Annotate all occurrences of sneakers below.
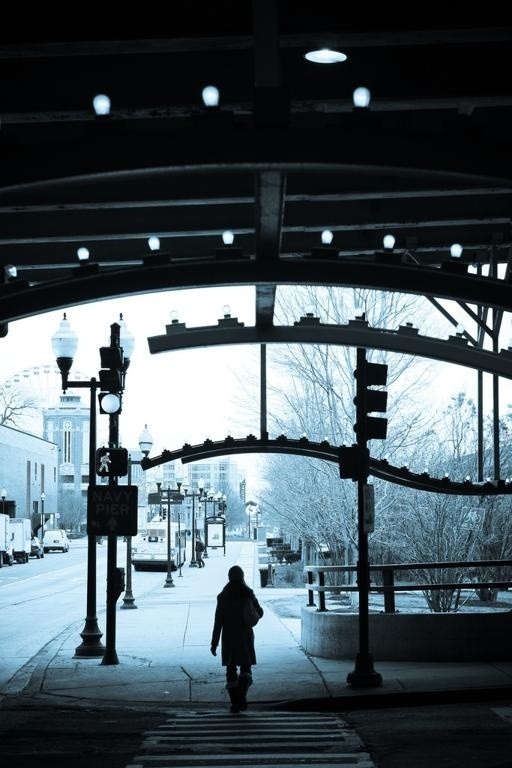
[197,563,206,569]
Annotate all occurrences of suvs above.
[42,529,70,553]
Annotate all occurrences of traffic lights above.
[95,448,128,476]
[99,345,123,415]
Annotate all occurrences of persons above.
[211,564,264,713]
[195,536,205,568]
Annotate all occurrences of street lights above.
[1,487,7,513]
[40,491,47,538]
[120,423,228,609]
[52,310,130,660]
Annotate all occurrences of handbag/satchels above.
[244,586,259,628]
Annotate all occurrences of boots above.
[238,673,254,712]
[226,679,242,713]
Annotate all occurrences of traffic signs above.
[363,484,374,533]
[459,507,485,531]
[87,484,138,537]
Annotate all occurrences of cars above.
[30,536,44,559]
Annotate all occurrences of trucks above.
[0,513,31,569]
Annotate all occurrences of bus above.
[130,521,192,572]
